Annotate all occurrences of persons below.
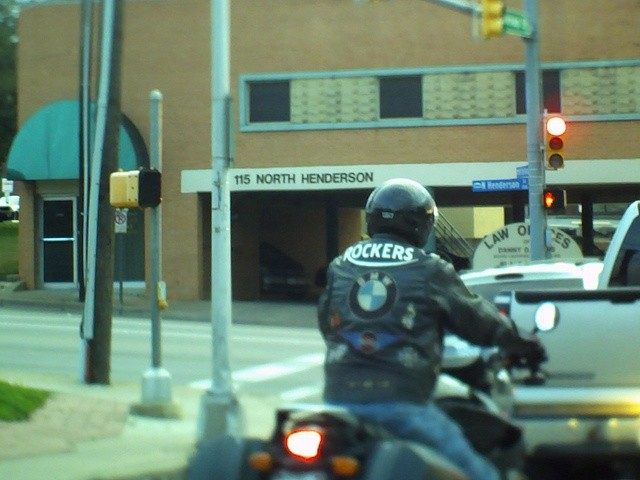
[316,179,547,480]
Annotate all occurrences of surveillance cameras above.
[1,177,14,205]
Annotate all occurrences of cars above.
[441,255,604,373]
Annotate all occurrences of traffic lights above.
[481,0,504,38]
[543,187,558,210]
[543,112,566,170]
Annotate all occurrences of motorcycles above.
[244,301,562,480]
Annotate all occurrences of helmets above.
[366,177,437,247]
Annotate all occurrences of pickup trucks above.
[489,199,640,458]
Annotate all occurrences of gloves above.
[506,326,548,370]
